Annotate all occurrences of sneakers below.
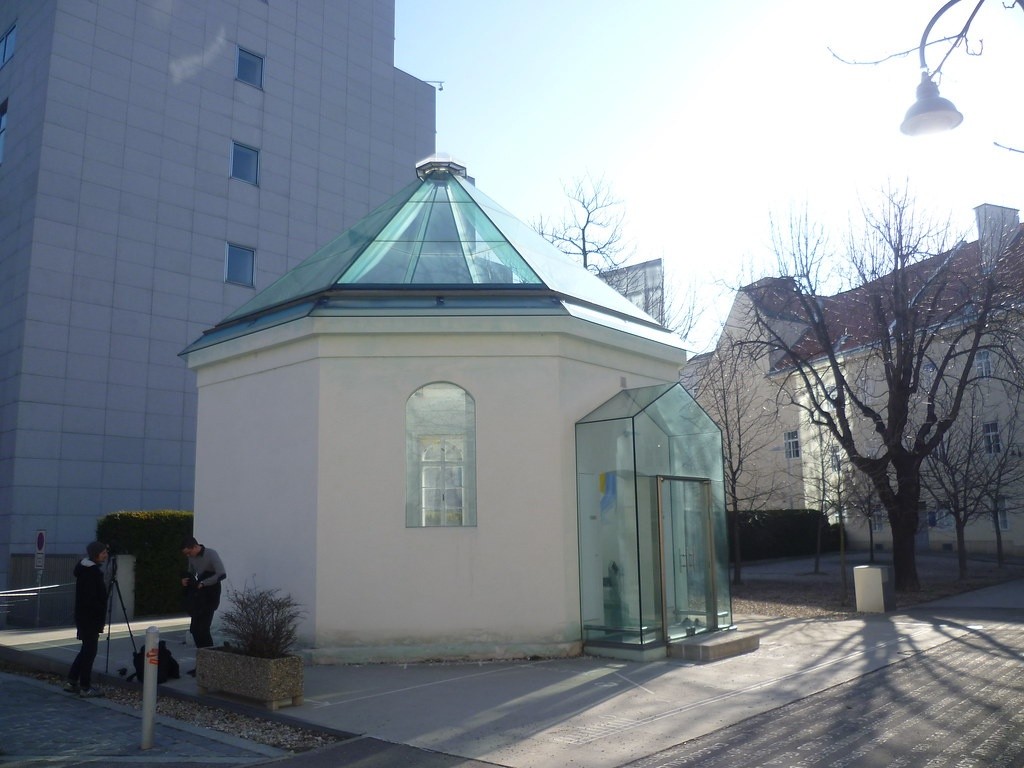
[63,683,81,692]
[80,688,104,698]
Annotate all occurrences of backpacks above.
[125,640,180,685]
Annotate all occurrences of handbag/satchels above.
[180,577,206,616]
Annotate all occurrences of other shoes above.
[187,669,196,677]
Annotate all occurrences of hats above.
[86,541,106,559]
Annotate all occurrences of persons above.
[180,536,226,678]
[63,541,105,697]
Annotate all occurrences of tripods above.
[107,577,137,674]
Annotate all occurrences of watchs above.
[201,581,204,586]
